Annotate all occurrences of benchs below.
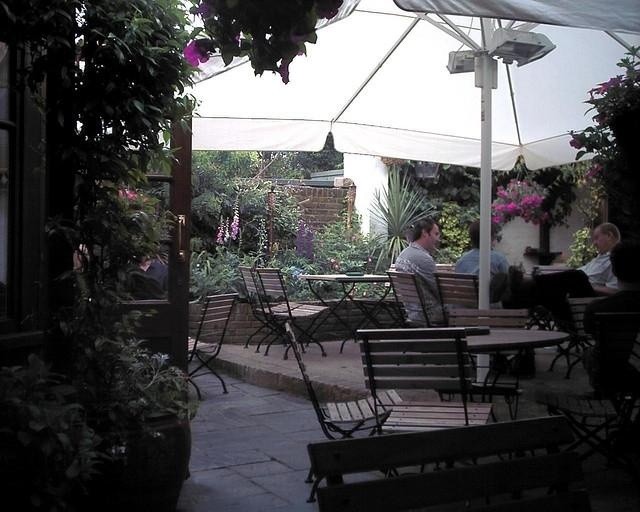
[306,416,591,512]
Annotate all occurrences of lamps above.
[488,28,556,67]
[446,50,474,74]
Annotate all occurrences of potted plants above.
[0,183,198,512]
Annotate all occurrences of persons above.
[453,216,514,310]
[582,238,640,400]
[395,218,450,328]
[484,221,623,303]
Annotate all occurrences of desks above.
[304,273,391,355]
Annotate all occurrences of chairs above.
[188,293,239,399]
[356,268,640,491]
[239,265,330,361]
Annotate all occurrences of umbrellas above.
[75,0,640,390]
